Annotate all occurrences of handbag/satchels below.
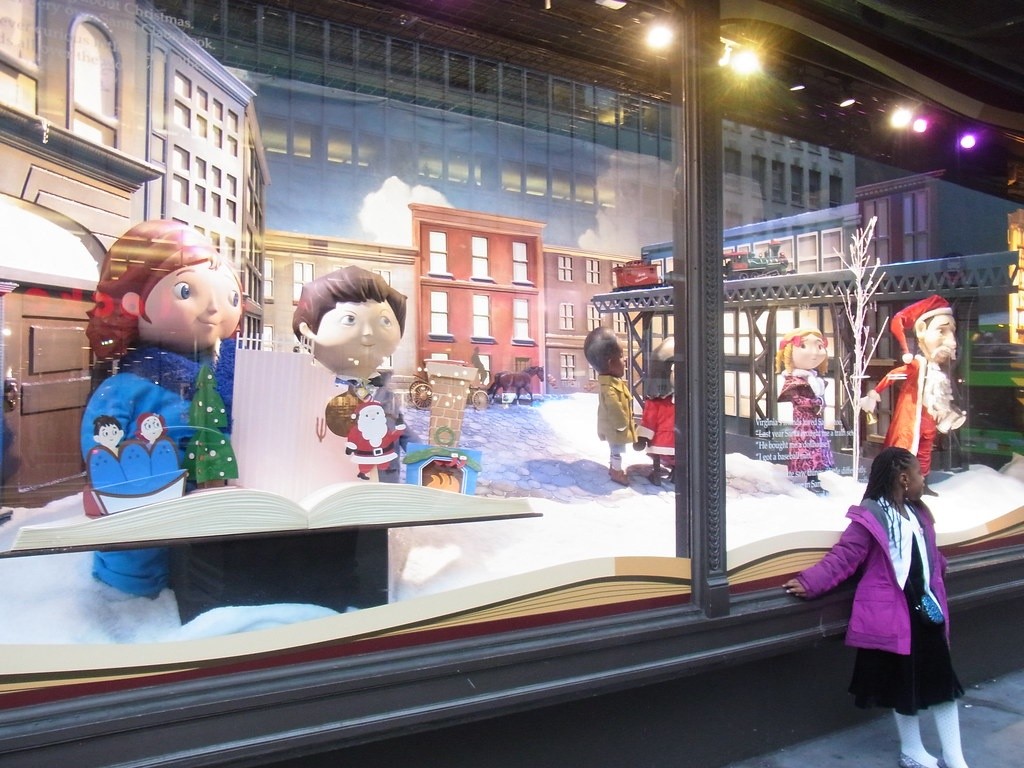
[922,594,943,624]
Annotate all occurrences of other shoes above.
[900,752,947,768]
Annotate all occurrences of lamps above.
[786,70,806,91]
[836,81,856,107]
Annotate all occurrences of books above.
[1,479,546,561]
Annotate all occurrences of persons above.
[584,325,639,486]
[632,335,676,487]
[292,263,425,482]
[78,218,244,603]
[774,325,833,497]
[858,294,968,497]
[783,445,970,768]
